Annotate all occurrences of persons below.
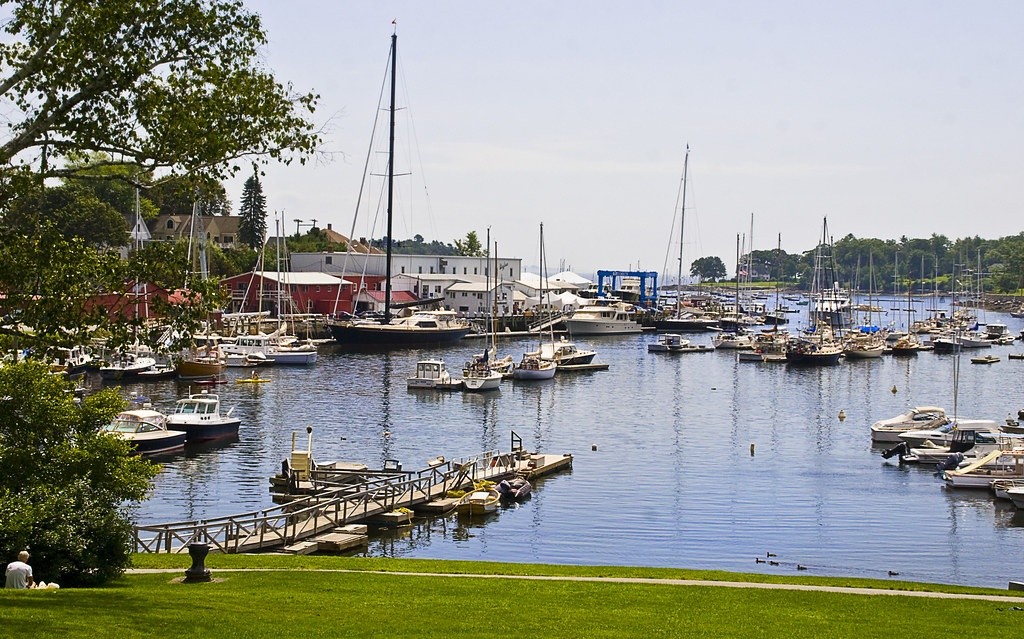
[5,551,36,588]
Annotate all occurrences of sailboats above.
[643,144,1024,365]
[0,170,317,404]
[465,221,610,391]
[325,19,471,349]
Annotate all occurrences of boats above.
[872,405,1024,513]
[456,476,535,516]
[406,358,463,391]
[564,295,649,337]
[93,386,241,464]
[268,427,371,503]
